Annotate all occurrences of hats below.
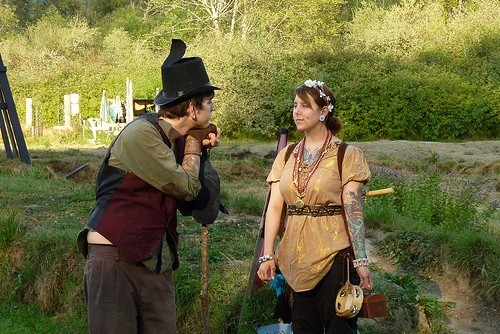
[155,39,222,105]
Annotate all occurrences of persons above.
[257,80,373,334]
[75,38,229,334]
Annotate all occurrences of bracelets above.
[258,255,274,262]
[352,258,369,268]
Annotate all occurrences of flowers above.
[304,78,334,113]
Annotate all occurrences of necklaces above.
[292,129,332,209]
